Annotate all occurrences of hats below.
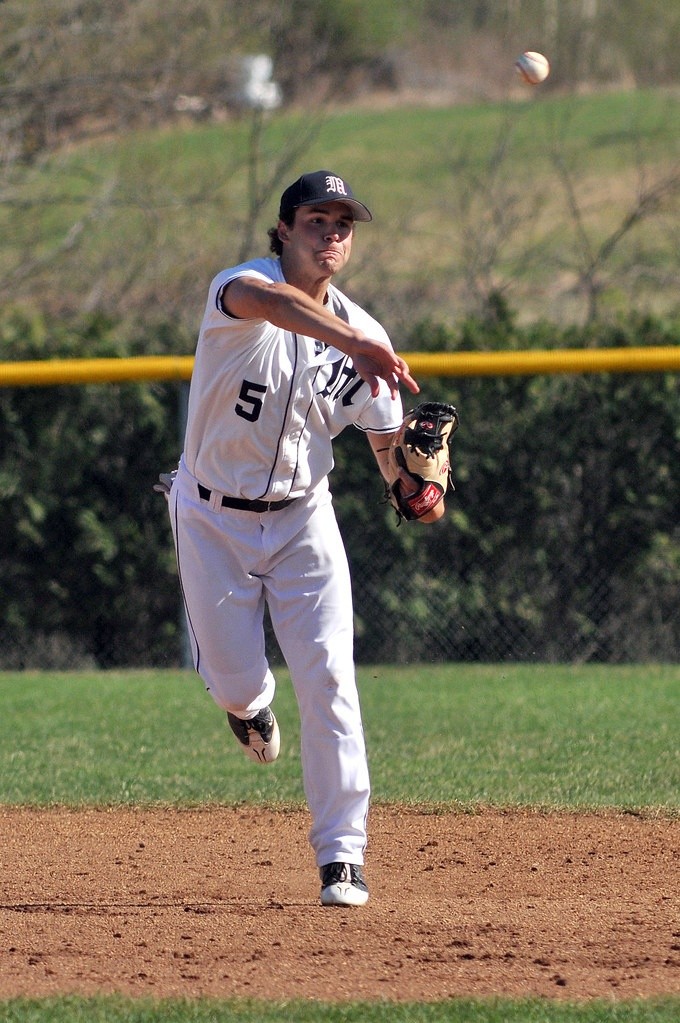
[280,171,374,222]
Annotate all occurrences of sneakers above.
[226,706,281,763]
[320,861,371,906]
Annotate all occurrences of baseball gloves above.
[374,400,460,529]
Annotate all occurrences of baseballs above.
[515,51,551,85]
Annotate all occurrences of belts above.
[198,482,296,514]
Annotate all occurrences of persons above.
[153,170,455,906]
